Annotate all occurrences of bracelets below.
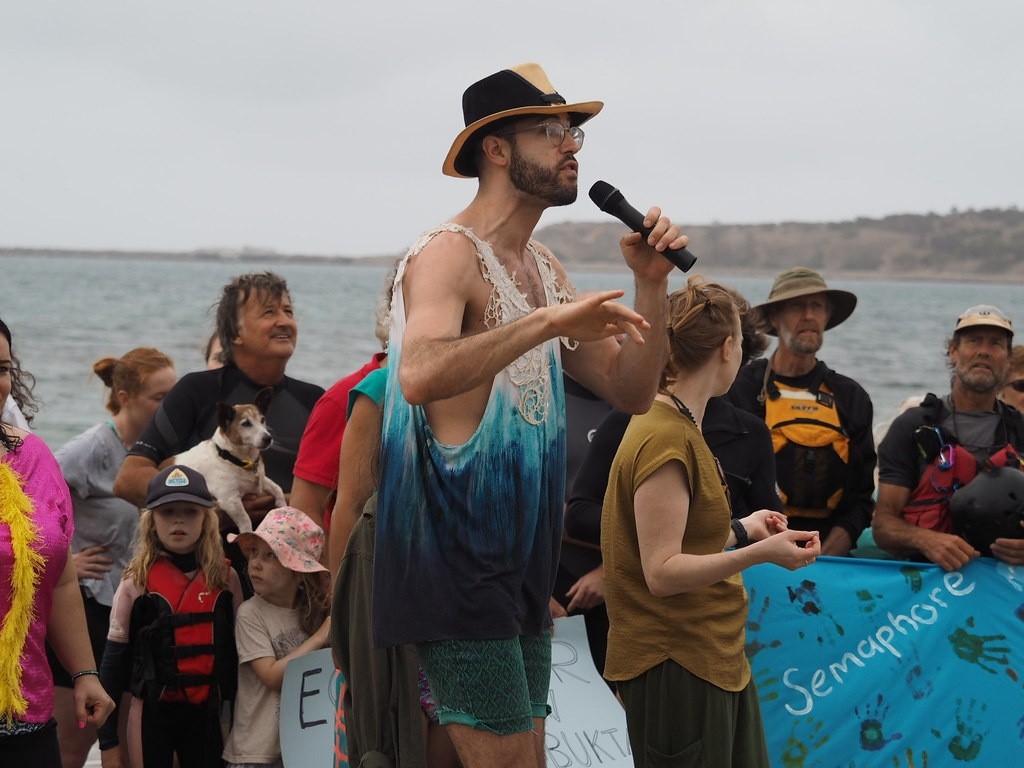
[71,670,100,682]
[731,517,749,549]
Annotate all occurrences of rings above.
[805,560,808,567]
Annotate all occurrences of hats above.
[953,304,1014,334]
[228,506,331,572]
[442,62,604,179]
[750,267,857,337]
[146,465,212,511]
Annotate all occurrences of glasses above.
[498,122,585,151]
[1004,379,1024,392]
[952,396,1008,455]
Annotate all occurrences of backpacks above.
[754,380,850,532]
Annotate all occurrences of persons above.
[0,64,1024,768]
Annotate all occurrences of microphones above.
[590,180,697,272]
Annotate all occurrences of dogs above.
[158,386,287,535]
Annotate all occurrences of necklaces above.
[666,389,733,517]
